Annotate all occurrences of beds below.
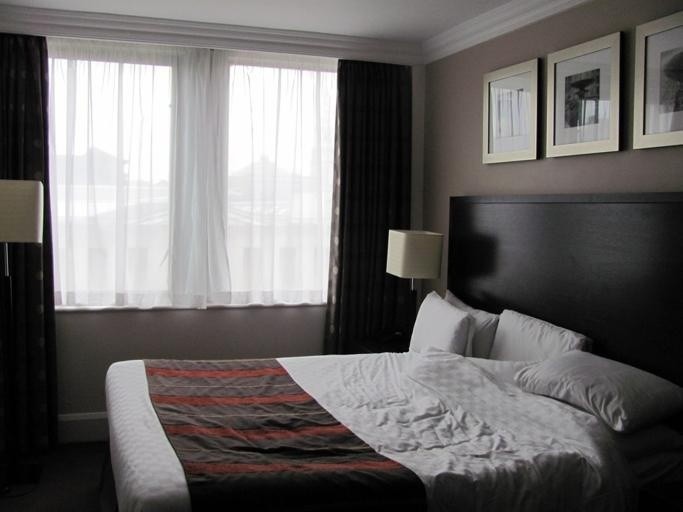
[104,193,683,510]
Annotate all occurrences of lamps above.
[387,228,443,315]
[0,178,44,309]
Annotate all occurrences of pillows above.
[408,290,473,356]
[516,350,682,431]
[443,291,499,359]
[487,309,588,368]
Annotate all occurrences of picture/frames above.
[545,32,621,158]
[631,8,683,150]
[482,58,539,164]
